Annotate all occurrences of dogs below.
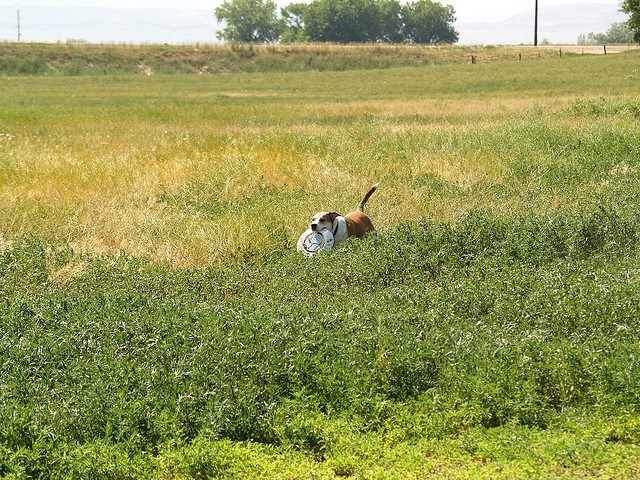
[311,183,381,248]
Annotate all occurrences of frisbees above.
[297,229,334,256]
